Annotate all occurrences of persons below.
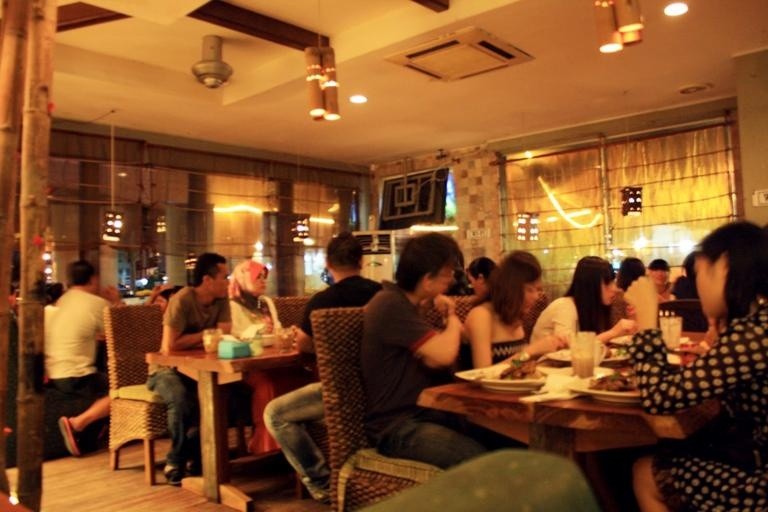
[531,257,636,361]
[40,284,67,386]
[647,258,675,302]
[618,255,662,332]
[468,255,497,294]
[46,259,111,456]
[621,220,768,512]
[358,232,490,472]
[226,259,311,453]
[459,250,569,388]
[670,252,708,333]
[261,233,384,507]
[146,252,233,487]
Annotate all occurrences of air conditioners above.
[351,229,394,283]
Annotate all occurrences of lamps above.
[46,280,52,284]
[44,267,52,274]
[289,149,311,238]
[102,110,126,242]
[307,81,325,121]
[156,216,167,234]
[324,86,340,123]
[46,276,53,279]
[118,172,128,178]
[664,2,689,17]
[621,91,643,217]
[322,1,338,84]
[213,151,603,234]
[41,252,52,261]
[593,0,624,54]
[623,33,643,44]
[304,0,322,88]
[612,0,644,33]
[184,252,199,270]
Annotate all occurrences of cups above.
[203,329,223,352]
[276,329,293,352]
[572,332,607,378]
[660,317,682,350]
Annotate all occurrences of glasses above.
[440,270,455,278]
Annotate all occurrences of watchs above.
[518,350,531,360]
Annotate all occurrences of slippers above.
[60,416,82,455]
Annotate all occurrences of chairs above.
[310,306,446,512]
[521,292,548,344]
[104,304,179,487]
[260,296,309,327]
[353,450,601,512]
[657,298,708,332]
[424,295,477,333]
[296,419,325,499]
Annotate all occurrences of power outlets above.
[752,189,768,207]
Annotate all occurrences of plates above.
[570,386,641,402]
[241,335,276,346]
[544,349,631,362]
[609,335,633,345]
[454,367,547,392]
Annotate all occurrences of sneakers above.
[186,462,200,475]
[165,467,182,484]
